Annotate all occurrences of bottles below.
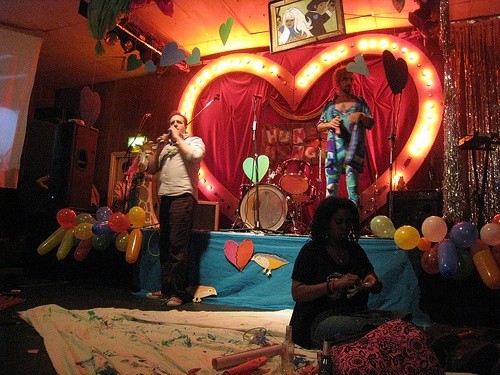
[319,339,333,375]
[280,324,297,375]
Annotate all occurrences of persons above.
[316,66,374,239]
[290,195,463,366]
[144,114,206,307]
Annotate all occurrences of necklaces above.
[329,243,349,263]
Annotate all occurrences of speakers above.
[192,200,220,232]
[49,122,99,209]
[387,189,443,237]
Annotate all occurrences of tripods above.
[220,98,284,234]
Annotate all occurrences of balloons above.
[369,214,500,290]
[38,206,147,263]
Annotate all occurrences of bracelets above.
[326,279,336,295]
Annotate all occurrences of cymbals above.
[303,133,328,148]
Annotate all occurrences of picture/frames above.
[107,151,150,207]
[268,0,346,53]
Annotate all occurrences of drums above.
[288,185,317,202]
[279,159,312,194]
[239,183,296,234]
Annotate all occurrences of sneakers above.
[146,291,164,299]
[161,297,183,306]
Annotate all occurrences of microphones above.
[253,94,262,99]
[144,113,151,117]
